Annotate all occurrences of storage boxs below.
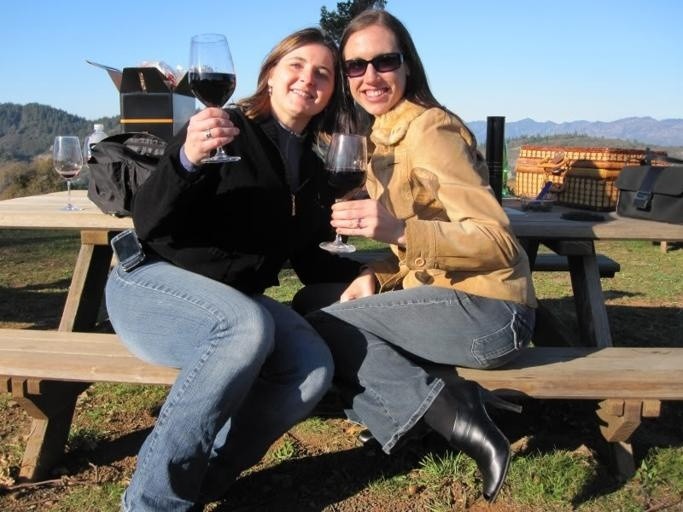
[84,59,201,153]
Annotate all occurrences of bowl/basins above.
[519,192,558,211]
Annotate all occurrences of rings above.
[355,218,362,228]
[205,130,212,140]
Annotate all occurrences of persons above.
[102,29,362,512]
[293,10,541,502]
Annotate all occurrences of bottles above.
[84,123,109,164]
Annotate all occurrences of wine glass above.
[52,134,86,213]
[189,33,242,165]
[319,132,368,253]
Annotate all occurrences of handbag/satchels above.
[87,131,169,217]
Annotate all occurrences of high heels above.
[448,380,523,505]
[359,422,430,449]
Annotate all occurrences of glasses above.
[342,52,404,78]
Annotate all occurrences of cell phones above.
[110,229,147,273]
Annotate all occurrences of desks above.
[0,190,683,461]
